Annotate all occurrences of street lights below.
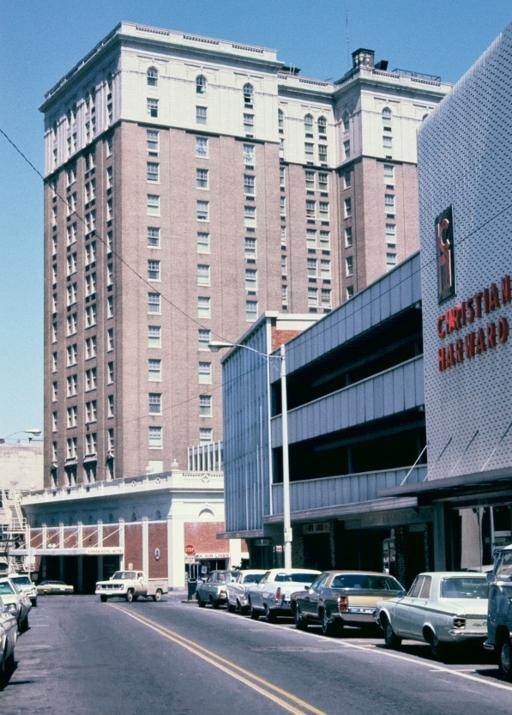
[208,340,293,568]
[0,428,42,443]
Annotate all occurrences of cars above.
[0,574,38,675]
[37,580,75,595]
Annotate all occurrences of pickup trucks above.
[95,570,168,603]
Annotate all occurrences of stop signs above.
[184,544,196,557]
